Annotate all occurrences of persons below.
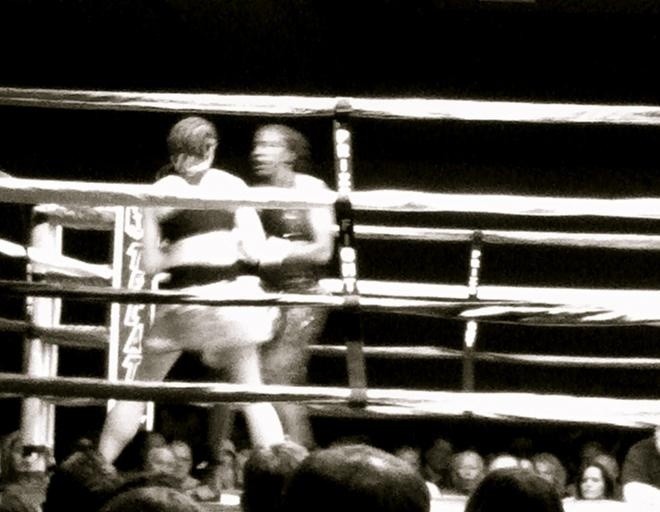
[209,122,337,454]
[1,428,659,512]
[96,113,311,468]
